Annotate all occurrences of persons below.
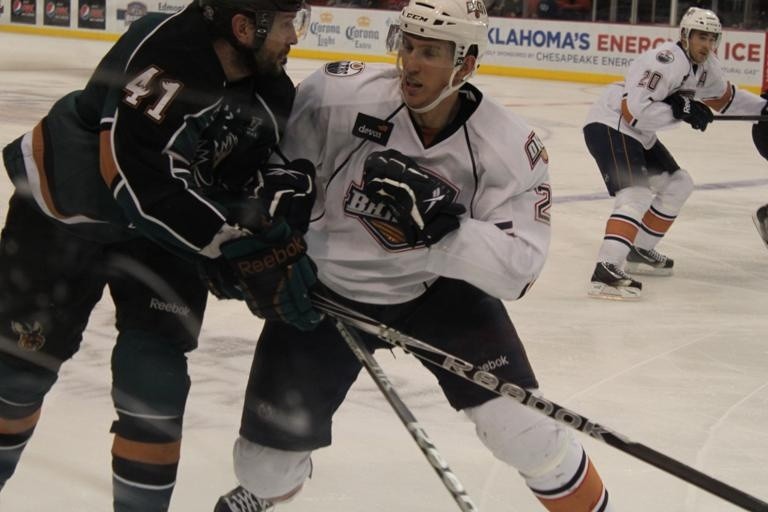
[0,0,322,512]
[213,0,616,512]
[583,8,768,291]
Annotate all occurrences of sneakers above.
[214,482,302,512]
[592,262,641,290]
[757,203,767,244]
[627,245,673,268]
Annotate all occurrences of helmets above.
[679,7,722,51]
[198,0,301,52]
[395,0,490,91]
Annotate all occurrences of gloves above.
[664,96,713,131]
[362,149,466,247]
[198,158,324,332]
